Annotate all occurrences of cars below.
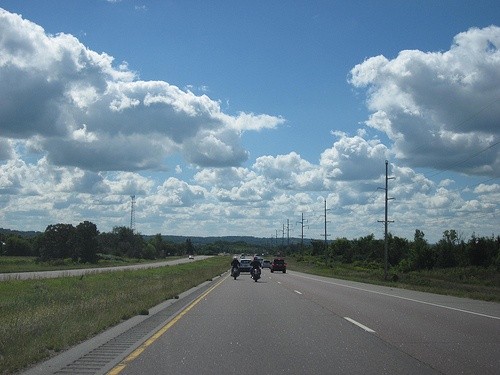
[239,255,252,271]
[258,259,271,269]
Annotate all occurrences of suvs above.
[271,259,287,273]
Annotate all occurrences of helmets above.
[254,257,258,261]
[234,257,237,260]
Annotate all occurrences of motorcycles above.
[231,263,240,280]
[250,264,261,282]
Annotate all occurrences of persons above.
[231,256,240,274]
[250,257,261,279]
